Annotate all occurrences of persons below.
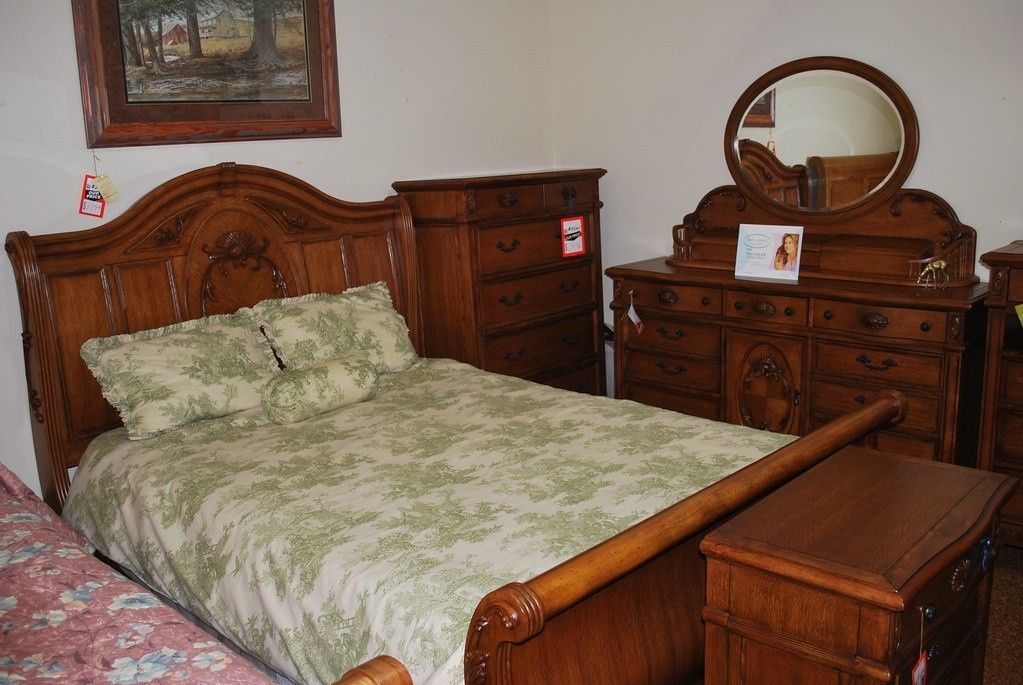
[769,233,799,272]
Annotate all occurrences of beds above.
[5,161,910,684]
[1,465,412,684]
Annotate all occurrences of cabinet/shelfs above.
[391,168,608,396]
[700,444,1020,684]
[604,184,989,467]
[975,241,1023,551]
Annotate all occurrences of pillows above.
[254,280,427,374]
[261,357,380,423]
[80,307,283,442]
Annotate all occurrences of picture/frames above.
[71,0,343,150]
[741,88,776,128]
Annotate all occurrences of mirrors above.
[723,56,919,224]
